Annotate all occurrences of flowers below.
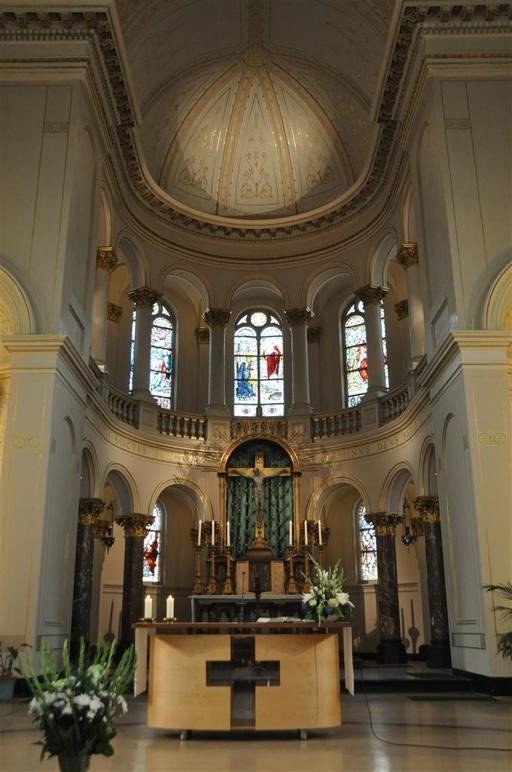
[300,553,356,628]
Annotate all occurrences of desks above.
[137,621,354,740]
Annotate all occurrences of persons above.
[230,467,289,528]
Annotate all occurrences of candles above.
[197,520,201,545]
[304,520,308,546]
[166,595,175,618]
[288,520,293,545]
[318,520,322,545]
[144,594,152,618]
[211,520,215,545]
[227,522,231,546]
